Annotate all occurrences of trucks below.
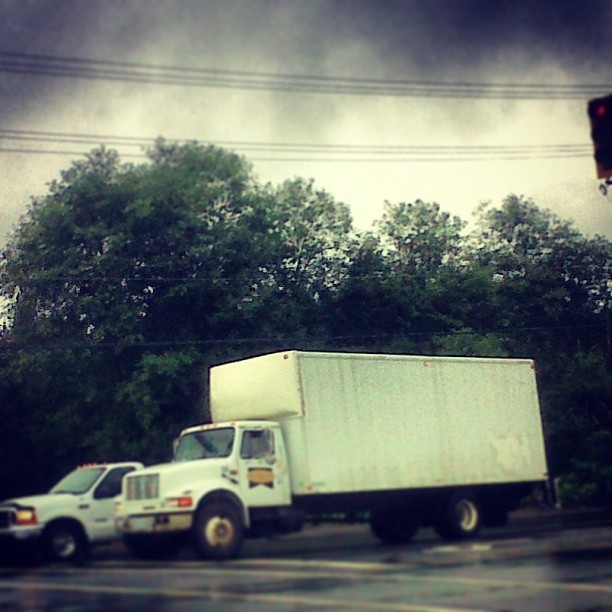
[119,350,548,562]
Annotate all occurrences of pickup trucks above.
[0,462,196,562]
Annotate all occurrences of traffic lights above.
[589,93,612,178]
